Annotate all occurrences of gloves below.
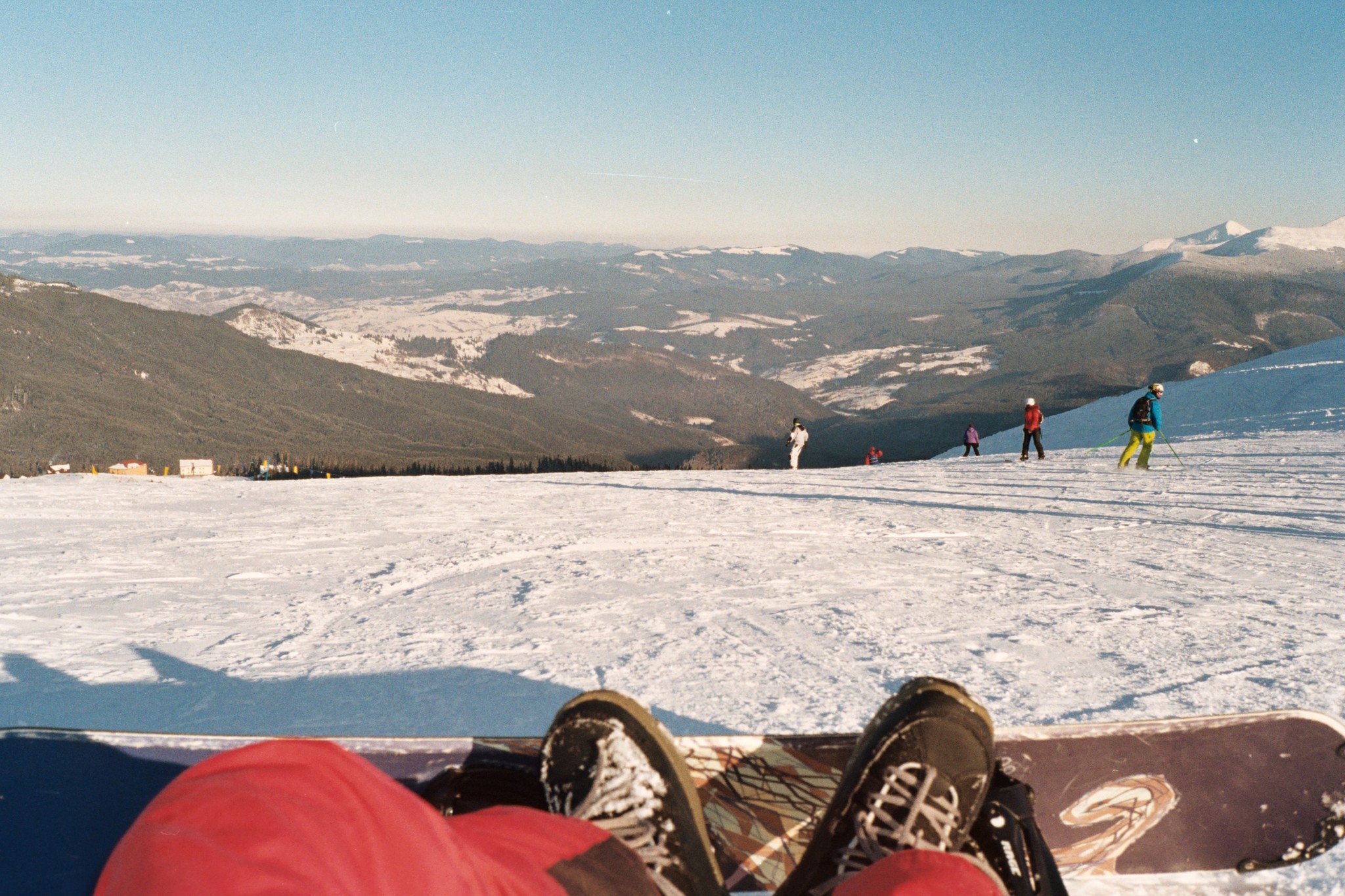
[785,443,788,447]
[964,443,966,446]
[804,445,807,448]
[976,443,979,448]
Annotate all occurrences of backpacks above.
[1129,395,1157,425]
[1036,409,1044,424]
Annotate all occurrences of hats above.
[1148,383,1164,395]
[969,424,974,428]
[793,418,799,424]
[1026,398,1035,405]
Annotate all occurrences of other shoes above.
[1020,454,1029,460]
[1039,455,1044,460]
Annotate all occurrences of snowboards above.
[0,698,1345,896]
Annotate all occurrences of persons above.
[866,447,883,465]
[963,424,980,457]
[1020,398,1046,461]
[98,678,1010,896]
[785,418,808,469]
[1117,382,1164,471]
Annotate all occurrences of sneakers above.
[808,677,995,895]
[538,691,728,896]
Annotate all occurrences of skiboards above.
[1003,458,1052,465]
[1110,463,1192,474]
